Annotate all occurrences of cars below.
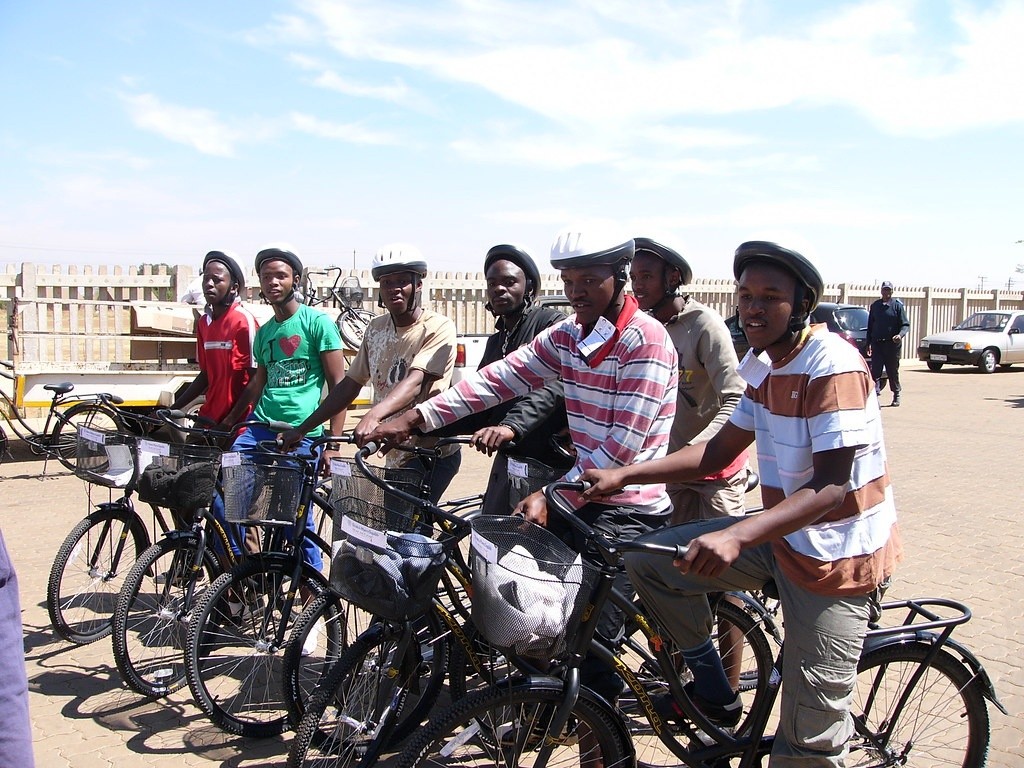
[915,309,1024,374]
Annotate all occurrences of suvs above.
[721,301,890,391]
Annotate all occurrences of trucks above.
[8,273,375,445]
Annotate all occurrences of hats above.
[881,281,894,291]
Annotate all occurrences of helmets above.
[733,241,824,313]
[204,251,245,294]
[484,244,542,301]
[550,228,636,270]
[254,248,302,279]
[632,236,693,285]
[371,251,428,282]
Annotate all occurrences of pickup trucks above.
[448,293,578,389]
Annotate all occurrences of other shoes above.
[636,679,742,728]
[153,562,204,583]
[493,713,578,746]
[222,596,266,624]
[892,392,901,406]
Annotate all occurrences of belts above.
[874,338,891,342]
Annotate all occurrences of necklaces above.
[501,312,522,358]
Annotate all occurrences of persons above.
[212,249,348,621]
[1004,316,1024,333]
[145,250,262,582]
[272,240,461,630]
[574,241,903,768]
[392,242,577,670]
[865,281,911,407]
[357,230,676,768]
[630,233,749,744]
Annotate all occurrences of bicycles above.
[0,356,126,476]
[45,391,546,761]
[297,266,379,354]
[393,479,1013,768]
[285,439,785,768]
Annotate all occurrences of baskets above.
[329,496,457,622]
[76,421,143,488]
[135,436,224,509]
[339,276,364,304]
[470,514,599,663]
[220,451,307,526]
[330,457,422,518]
